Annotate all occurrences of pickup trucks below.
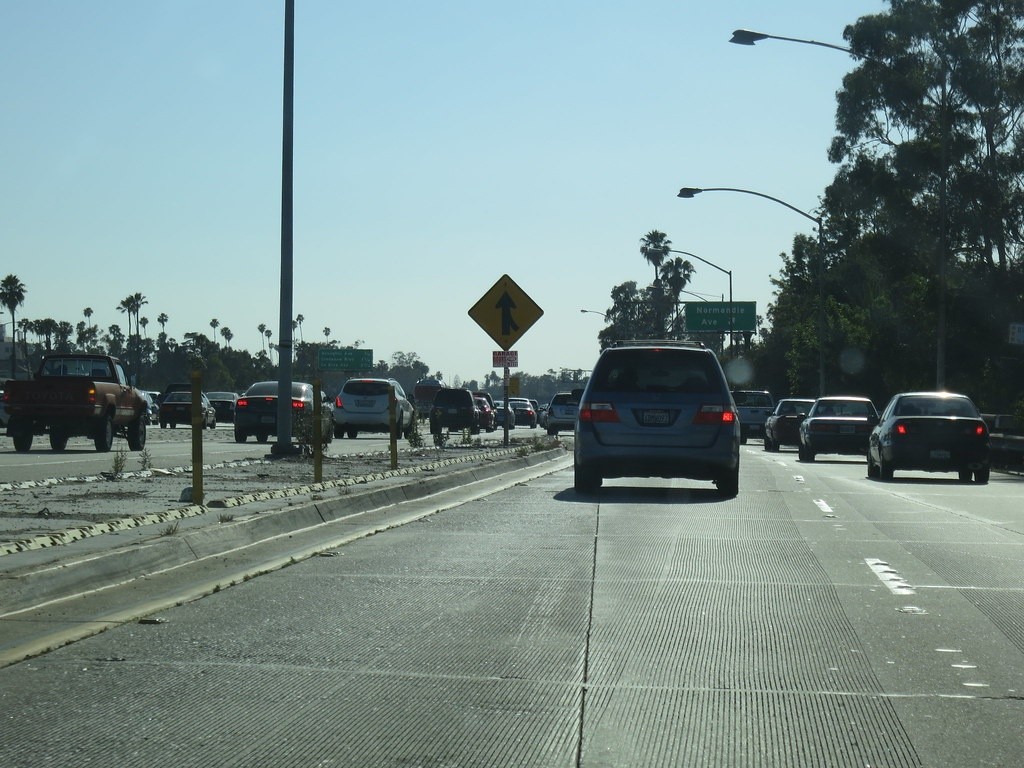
[730,390,777,444]
[3,351,149,454]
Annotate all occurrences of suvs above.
[572,340,748,499]
[429,387,481,435]
[331,376,415,440]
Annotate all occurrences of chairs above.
[754,397,766,407]
[821,407,836,416]
[607,369,634,392]
[900,403,922,415]
[781,406,796,416]
[734,394,748,406]
[92,368,106,377]
[851,406,867,416]
[50,364,67,376]
[676,376,711,393]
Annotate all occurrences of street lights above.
[649,246,732,394]
[677,186,827,398]
[729,29,947,392]
[646,285,725,367]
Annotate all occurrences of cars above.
[205,391,240,423]
[0,377,161,428]
[763,398,817,453]
[159,389,216,430]
[234,381,331,444]
[866,391,994,484]
[796,396,880,463]
[470,390,579,436]
